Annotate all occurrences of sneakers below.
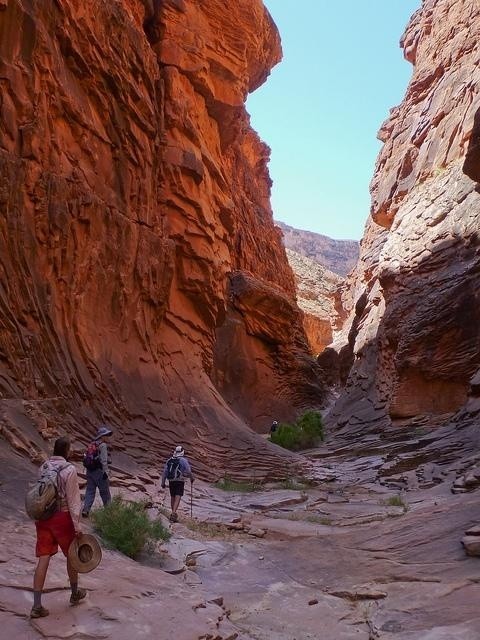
[170,512,178,521]
[30,605,49,617]
[70,589,86,604]
[82,509,90,518]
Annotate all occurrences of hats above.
[273,421,277,425]
[69,534,102,573]
[173,446,184,457]
[95,428,111,440]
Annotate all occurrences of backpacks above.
[25,461,72,520]
[83,441,103,468]
[167,457,182,479]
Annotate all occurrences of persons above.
[268,418,279,435]
[160,444,196,525]
[29,435,88,620]
[80,427,114,519]
